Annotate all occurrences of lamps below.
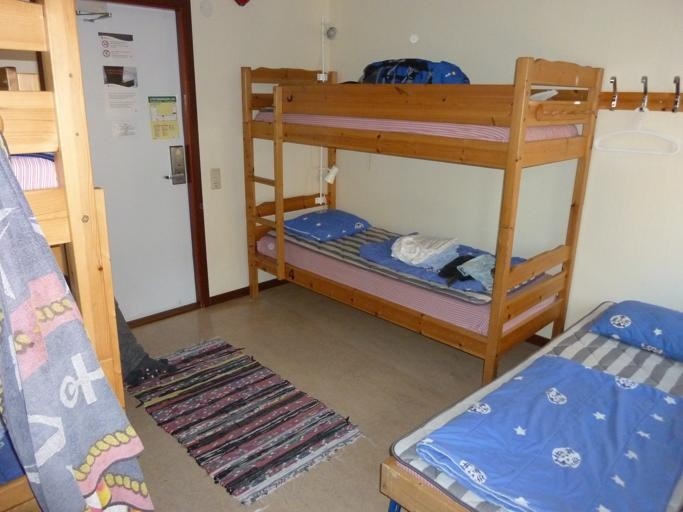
[318,165,339,204]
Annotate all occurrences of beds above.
[1,0,123,512]
[239,45,603,388]
[378,279,683,512]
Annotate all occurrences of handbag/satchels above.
[359,58,470,84]
[391,233,461,271]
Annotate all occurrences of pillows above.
[283,209,372,242]
[589,299,683,364]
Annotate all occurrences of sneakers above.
[127,358,176,384]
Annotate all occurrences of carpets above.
[124,336,365,508]
